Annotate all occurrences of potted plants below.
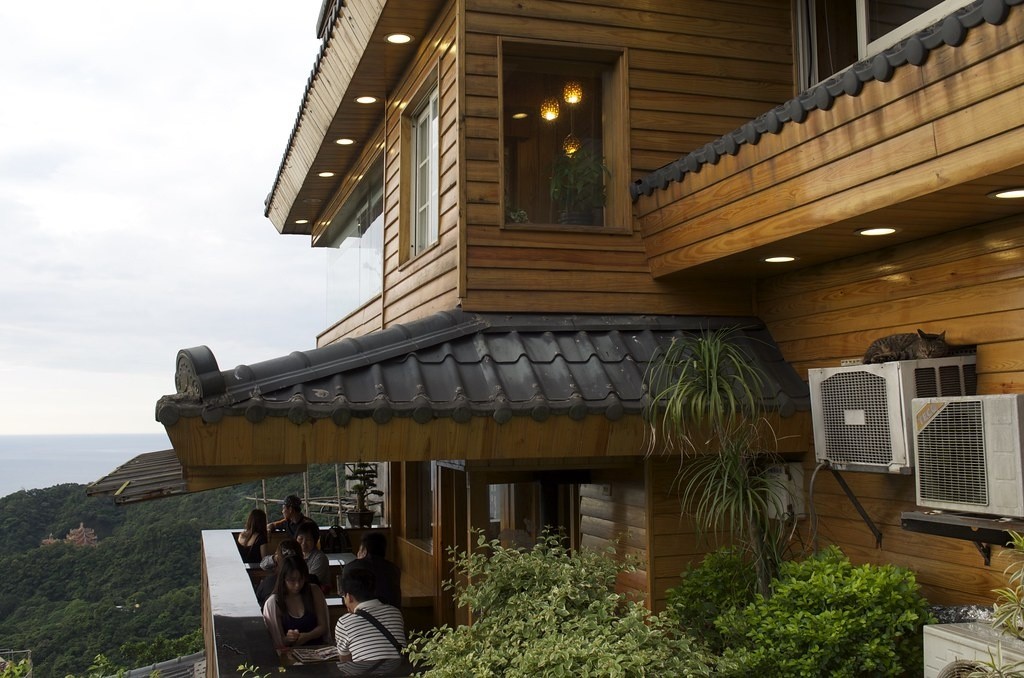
[338,461,384,529]
[544,148,612,226]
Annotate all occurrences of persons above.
[256,539,320,609]
[264,554,331,647]
[335,568,407,662]
[338,532,400,609]
[260,522,330,584]
[336,660,402,678]
[266,496,321,551]
[234,509,267,563]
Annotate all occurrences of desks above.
[244,551,358,584]
[281,644,347,678]
[324,597,348,621]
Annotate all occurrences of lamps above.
[541,78,584,158]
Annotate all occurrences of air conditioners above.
[908,393,1024,521]
[922,622,1024,678]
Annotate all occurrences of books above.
[292,646,338,663]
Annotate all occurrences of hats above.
[276,494,302,508]
